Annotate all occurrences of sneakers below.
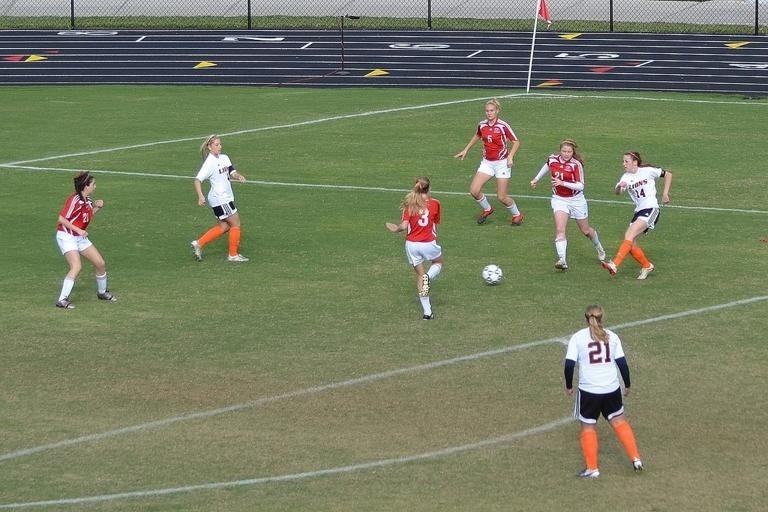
[633,458,644,472]
[227,254,249,262]
[96,289,116,301]
[190,240,202,261]
[422,312,435,321]
[56,298,76,309]
[420,273,431,297]
[575,468,599,480]
[477,208,653,278]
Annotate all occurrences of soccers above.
[482,264,502,285]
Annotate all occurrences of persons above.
[54,169,118,309]
[187,133,252,264]
[599,149,675,281]
[529,138,606,270]
[563,302,646,478]
[453,98,524,228]
[385,176,443,322]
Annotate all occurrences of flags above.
[537,0,552,29]
